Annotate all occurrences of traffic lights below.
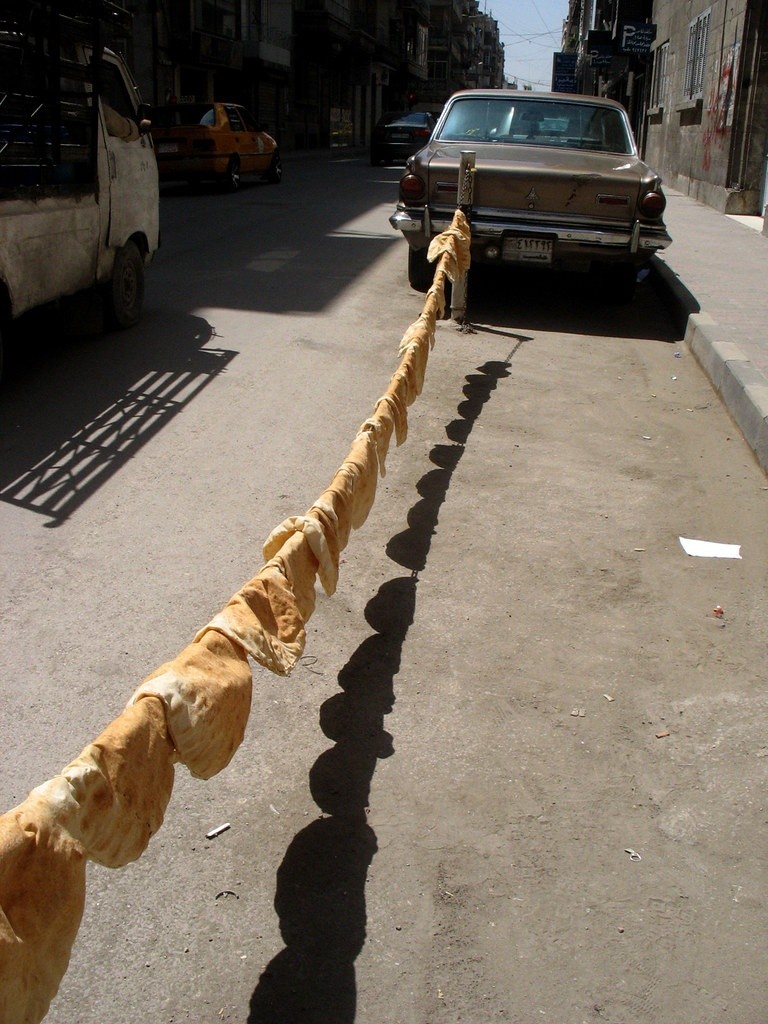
[409,94,417,107]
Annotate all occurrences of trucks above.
[1,0,177,333]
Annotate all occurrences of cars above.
[388,87,673,306]
[152,102,283,194]
[369,110,437,166]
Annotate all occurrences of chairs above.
[567,118,591,136]
